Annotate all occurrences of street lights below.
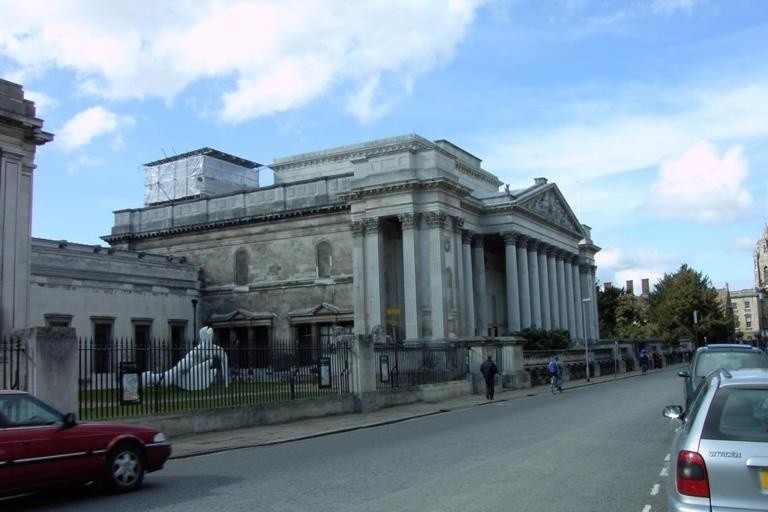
[190,298,198,350]
[580,297,592,383]
[727,330,733,343]
[703,336,707,345]
[758,286,767,344]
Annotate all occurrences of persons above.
[548,356,564,392]
[480,355,499,400]
[638,350,648,372]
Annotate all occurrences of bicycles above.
[639,364,647,375]
[549,367,565,396]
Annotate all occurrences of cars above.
[661,365,767,512]
[677,342,767,408]
[0,386,175,504]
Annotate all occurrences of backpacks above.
[550,363,556,374]
